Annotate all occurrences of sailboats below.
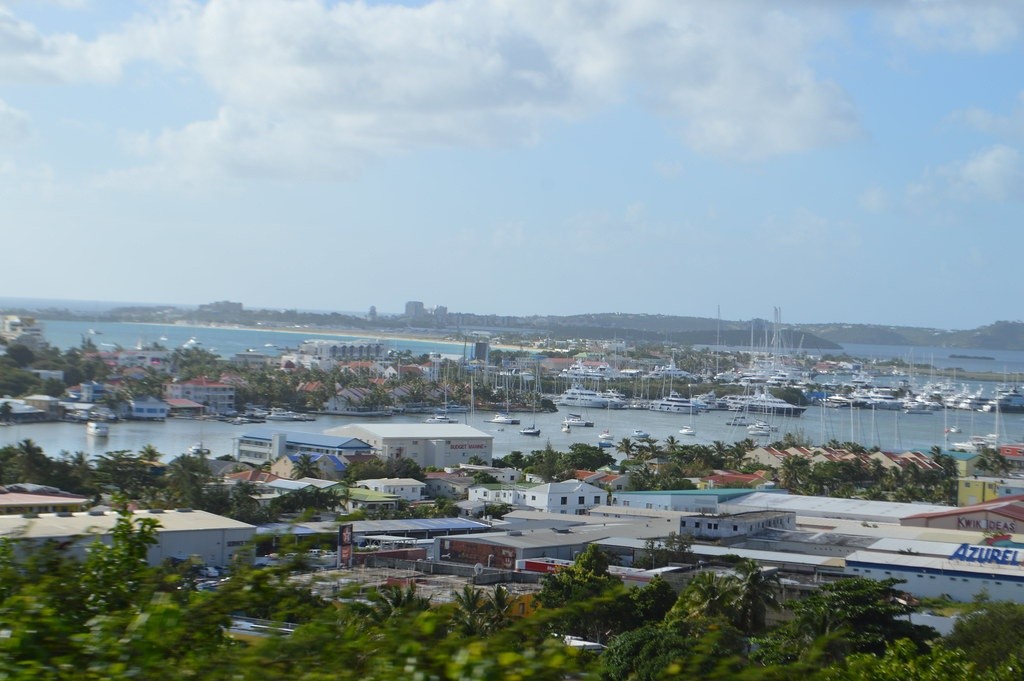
[726,375,780,437]
[562,376,594,427]
[519,384,540,435]
[679,394,697,436]
[599,399,615,440]
[483,377,520,425]
[423,377,459,424]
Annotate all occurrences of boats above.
[221,408,316,421]
[818,350,1024,415]
[550,307,807,416]
[631,428,649,438]
[562,423,571,432]
[591,441,614,449]
[87,422,109,437]
